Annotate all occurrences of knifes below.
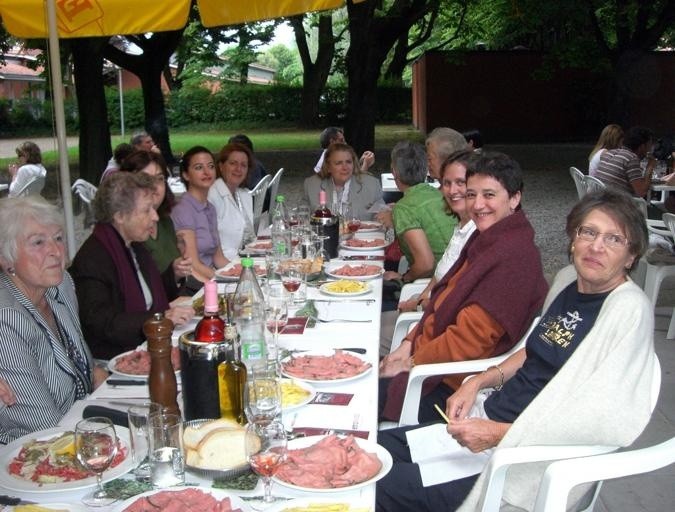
[106,379,184,386]
[1,495,37,506]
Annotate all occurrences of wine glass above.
[72,414,121,507]
[244,420,289,510]
[242,377,282,451]
[265,300,289,352]
[253,200,354,309]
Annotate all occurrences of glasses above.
[576,223,631,249]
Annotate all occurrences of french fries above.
[247,380,312,407]
[279,503,371,512]
[9,504,71,511]
[324,278,366,294]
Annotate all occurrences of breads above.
[181,418,262,472]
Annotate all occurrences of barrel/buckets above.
[309,213,339,260]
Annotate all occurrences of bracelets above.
[485,362,505,393]
[411,354,416,369]
[399,270,410,284]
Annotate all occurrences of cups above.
[127,398,168,482]
[149,414,185,486]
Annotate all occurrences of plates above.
[248,434,395,492]
[106,348,185,378]
[171,417,269,480]
[0,421,152,495]
[278,350,373,384]
[183,219,395,333]
[113,485,257,512]
[272,497,371,511]
[249,377,317,412]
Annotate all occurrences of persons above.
[0,195,113,445]
[379,153,550,427]
[375,126,472,228]
[118,149,209,300]
[165,145,231,282]
[594,126,657,198]
[588,123,625,193]
[377,151,489,359]
[204,143,253,265]
[0,375,18,409]
[295,143,390,234]
[130,130,162,155]
[229,133,274,210]
[65,167,197,362]
[385,139,461,300]
[312,127,376,175]
[99,142,138,183]
[659,152,675,213]
[376,191,658,512]
[7,140,48,198]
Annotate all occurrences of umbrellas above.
[0,0,363,260]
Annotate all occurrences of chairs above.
[397,315,620,512]
[267,168,284,212]
[582,175,607,200]
[569,168,586,202]
[663,211,674,342]
[389,311,422,356]
[634,199,673,327]
[533,435,673,509]
[16,177,44,198]
[78,182,98,204]
[248,174,271,236]
[398,255,432,282]
[398,284,437,309]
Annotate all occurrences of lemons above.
[48,435,83,457]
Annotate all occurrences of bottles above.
[231,255,268,377]
[195,280,224,342]
[314,191,333,218]
[218,325,247,422]
[271,196,293,257]
[142,312,183,450]
